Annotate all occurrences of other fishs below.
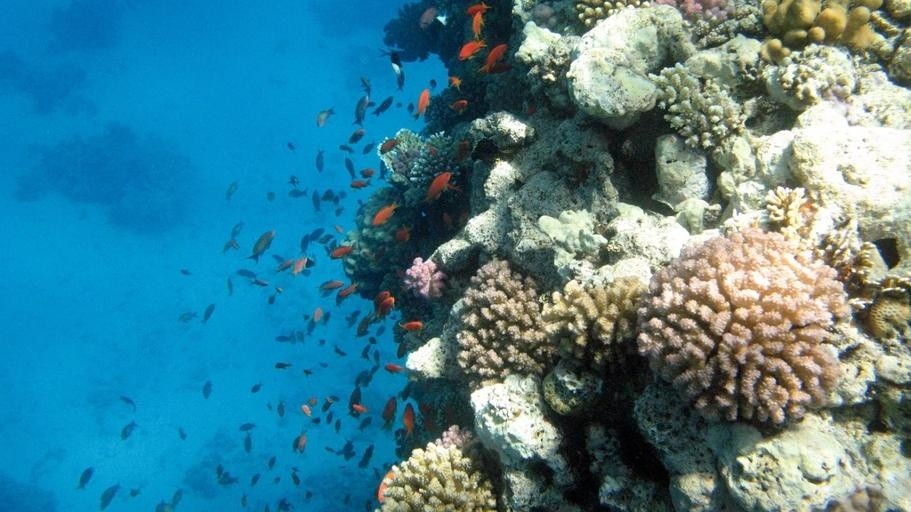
[176,5,509,512]
